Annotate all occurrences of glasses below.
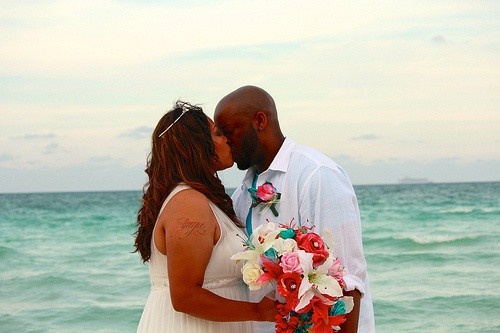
[158,106,190,138]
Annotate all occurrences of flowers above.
[248,181,281,217]
[230,218,355,333]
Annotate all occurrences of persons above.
[214,85,375,333]
[131,99,282,333]
